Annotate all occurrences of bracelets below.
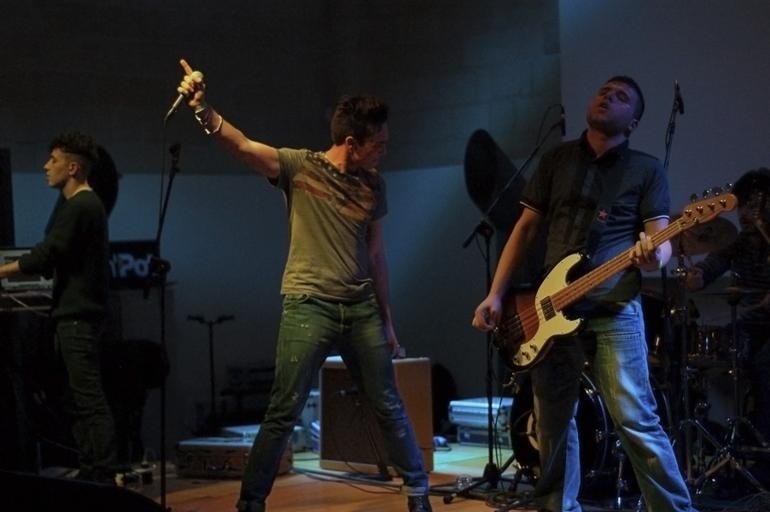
[194,106,224,136]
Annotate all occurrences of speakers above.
[319,357,434,475]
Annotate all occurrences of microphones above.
[675,81,686,115]
[559,107,568,136]
[164,71,203,120]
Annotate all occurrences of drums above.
[643,365,712,446]
[685,325,732,376]
[510,367,608,480]
[641,288,689,369]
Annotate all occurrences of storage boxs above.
[175,434,292,479]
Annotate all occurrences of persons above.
[0,128,121,483]
[684,166,769,498]
[470,74,698,512]
[175,56,435,511]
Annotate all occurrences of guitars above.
[495,184,738,372]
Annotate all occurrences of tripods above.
[603,312,770,512]
[444,236,531,505]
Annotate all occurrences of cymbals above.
[664,215,738,258]
